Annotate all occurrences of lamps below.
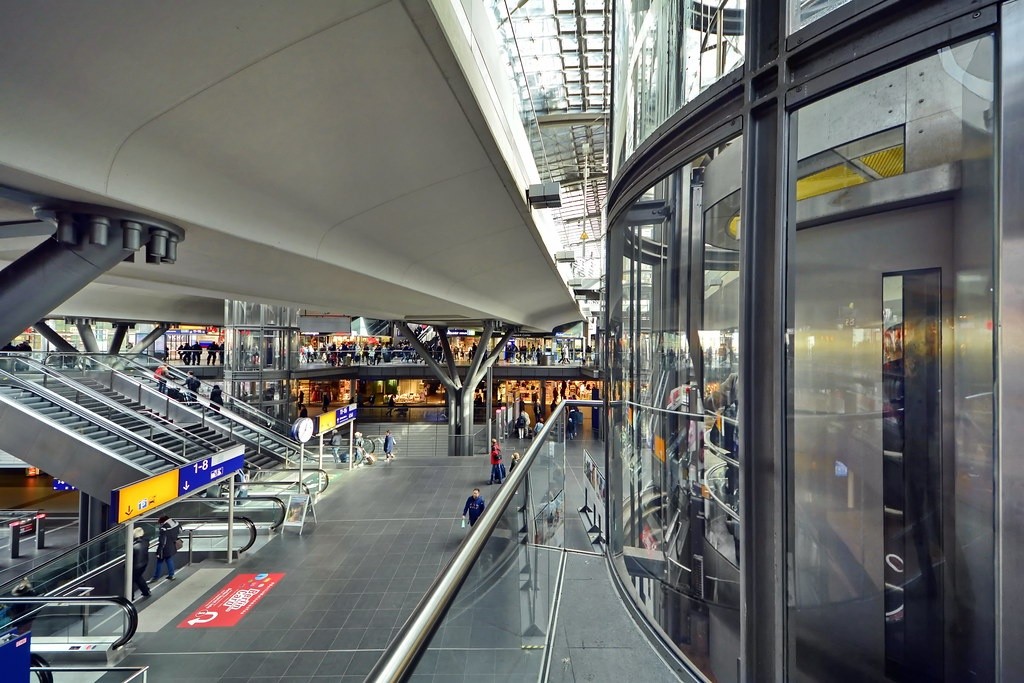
[148,228,168,257]
[567,278,582,286]
[79,319,85,325]
[162,234,178,261]
[120,221,142,251]
[55,211,77,245]
[85,319,91,325]
[72,318,79,324]
[525,182,563,209]
[158,322,179,328]
[87,214,111,246]
[554,251,576,262]
[121,252,135,263]
[146,244,160,265]
[64,317,71,324]
[91,319,96,325]
[112,322,136,329]
[482,318,522,332]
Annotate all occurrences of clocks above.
[295,417,314,443]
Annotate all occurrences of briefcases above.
[495,465,505,480]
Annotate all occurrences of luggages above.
[340,454,346,462]
[367,453,377,465]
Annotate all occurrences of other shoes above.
[141,591,151,595]
[166,575,176,579]
[384,458,390,462]
[390,454,395,460]
[146,577,158,583]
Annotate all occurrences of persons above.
[487,437,502,484]
[133,527,152,599]
[0,339,31,371]
[301,337,599,366]
[384,429,395,462]
[153,516,183,580]
[155,363,224,415]
[165,341,224,365]
[331,430,341,462]
[224,462,250,505]
[6,581,32,637]
[352,432,364,466]
[510,452,519,472]
[462,489,485,528]
[293,379,601,440]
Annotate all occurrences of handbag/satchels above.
[176,538,183,550]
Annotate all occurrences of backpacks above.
[494,448,502,461]
[191,376,201,388]
[154,367,163,379]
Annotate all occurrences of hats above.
[492,439,496,443]
[134,527,144,538]
